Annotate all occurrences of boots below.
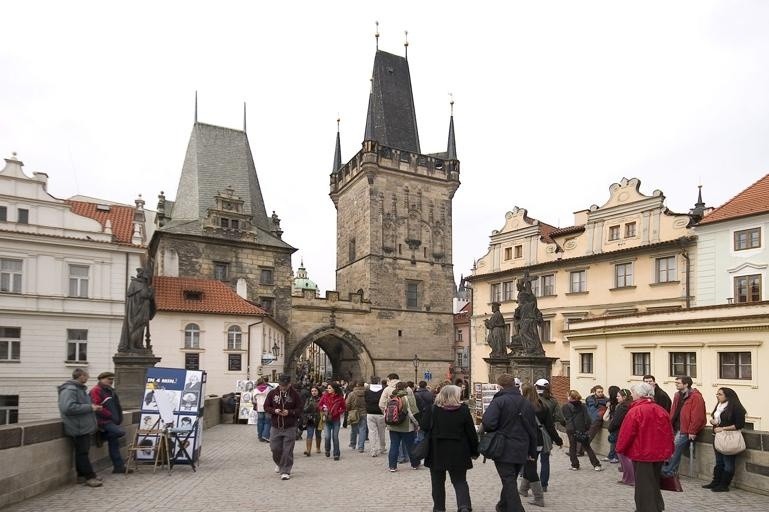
[527,481,544,507]
[711,469,732,492]
[702,468,723,489]
[304,439,312,456]
[518,478,530,496]
[316,437,321,452]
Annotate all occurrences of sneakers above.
[77,476,103,483]
[281,474,290,480]
[411,465,418,469]
[334,455,339,460]
[390,467,398,472]
[96,430,103,448]
[617,480,635,486]
[85,478,103,487]
[275,465,280,473]
[326,450,330,457]
[350,446,388,457]
[565,452,619,471]
[262,436,271,442]
[112,466,133,473]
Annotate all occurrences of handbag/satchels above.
[318,416,323,430]
[413,435,430,460]
[659,474,683,492]
[574,431,589,442]
[348,410,358,423]
[714,430,746,455]
[478,430,506,459]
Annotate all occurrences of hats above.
[279,374,291,385]
[513,378,520,387]
[98,372,117,379]
[534,378,549,386]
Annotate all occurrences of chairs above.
[125,427,164,475]
[164,420,199,473]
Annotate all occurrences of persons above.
[58,367,102,488]
[506,266,545,356]
[117,264,156,354]
[479,301,510,361]
[248,372,707,510]
[87,370,135,474]
[701,387,747,493]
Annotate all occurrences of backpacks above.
[608,432,618,442]
[385,394,406,424]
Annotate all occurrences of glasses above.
[675,382,684,384]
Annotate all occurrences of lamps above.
[411,354,420,385]
[262,343,280,365]
